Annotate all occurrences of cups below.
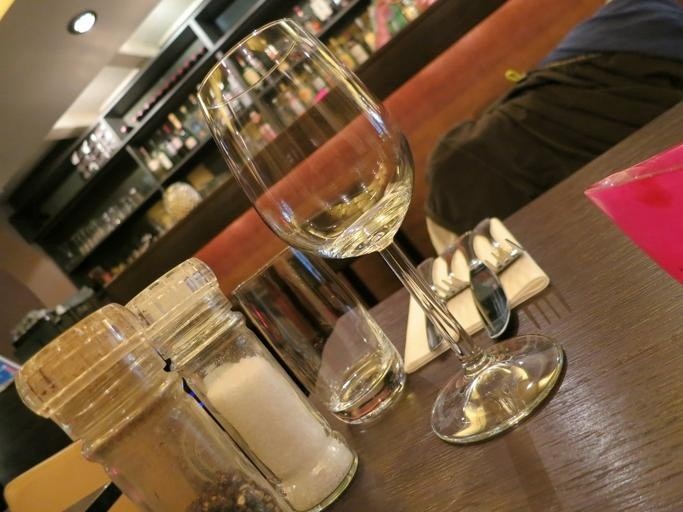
[232,242,408,427]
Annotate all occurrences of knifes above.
[454,235,513,339]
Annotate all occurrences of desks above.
[306,102,681,512]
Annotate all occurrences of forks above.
[471,218,520,274]
[430,243,471,300]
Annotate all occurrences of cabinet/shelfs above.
[5,0,431,274]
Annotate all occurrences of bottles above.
[1,0,446,294]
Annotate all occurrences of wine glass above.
[196,19,563,446]
